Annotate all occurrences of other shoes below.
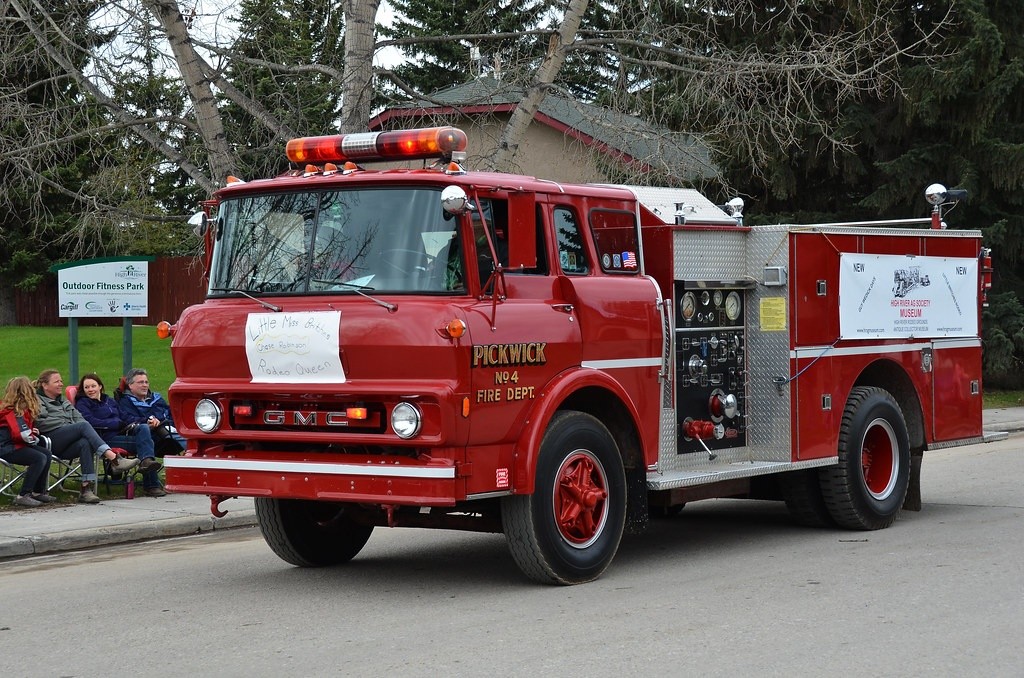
[77,481,100,503]
[141,487,167,497]
[137,457,161,473]
[109,453,141,476]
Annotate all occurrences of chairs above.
[0,386,188,506]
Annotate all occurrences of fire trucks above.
[154,123,995,588]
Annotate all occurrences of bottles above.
[126,475,134,500]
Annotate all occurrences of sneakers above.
[14,493,43,508]
[30,491,57,503]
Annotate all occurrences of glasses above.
[134,381,149,385]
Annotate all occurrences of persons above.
[75,374,166,497]
[119,368,187,449]
[0,377,57,506]
[33,371,140,503]
